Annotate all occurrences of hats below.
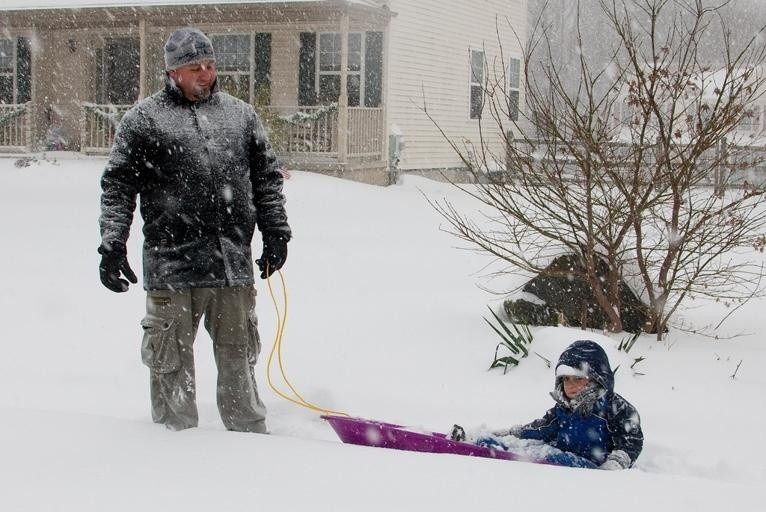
[555,362,590,377]
[164,29,215,70]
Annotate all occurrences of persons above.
[93,27,293,438]
[476,334,643,468]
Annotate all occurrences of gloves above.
[494,426,522,438]
[256,234,286,279]
[98,241,137,292]
[598,451,630,471]
